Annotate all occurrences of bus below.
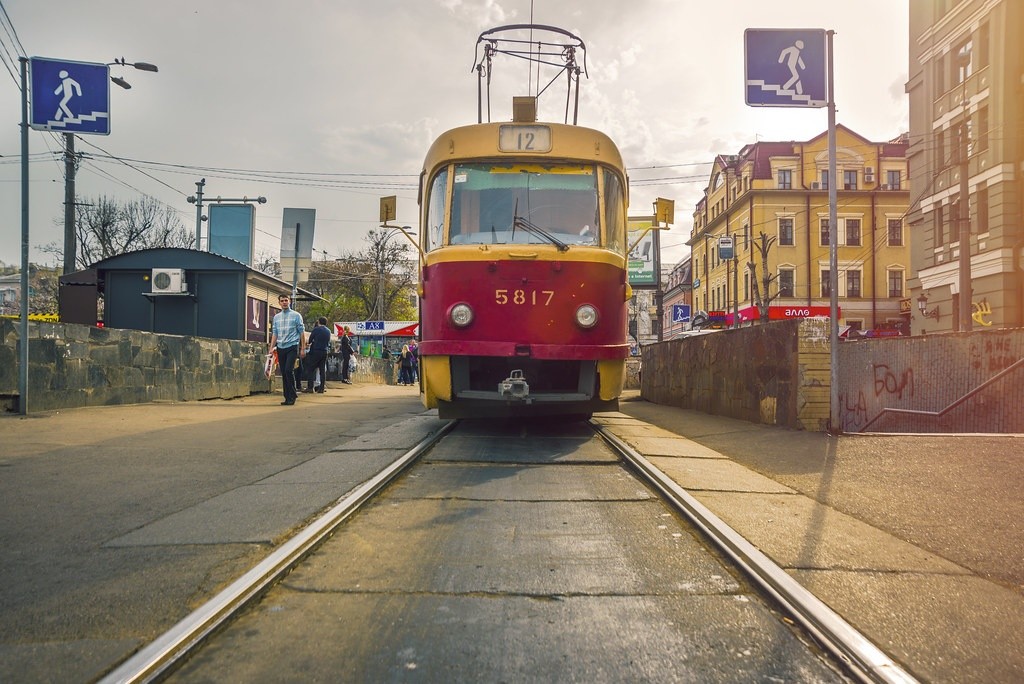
[379,96,676,424]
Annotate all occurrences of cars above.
[865,322,910,337]
[639,330,721,382]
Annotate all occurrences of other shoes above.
[281,395,298,405]
[301,388,313,393]
[410,384,414,386]
[404,384,407,386]
[317,390,323,393]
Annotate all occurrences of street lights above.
[63,62,158,272]
[704,232,738,329]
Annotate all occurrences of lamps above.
[916,293,940,323]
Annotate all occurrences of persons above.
[381,340,417,386]
[294,317,355,393]
[269,294,306,406]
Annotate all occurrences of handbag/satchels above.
[348,354,357,372]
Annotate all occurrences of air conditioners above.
[152,268,185,294]
[810,181,822,189]
[865,166,875,174]
[865,175,874,182]
